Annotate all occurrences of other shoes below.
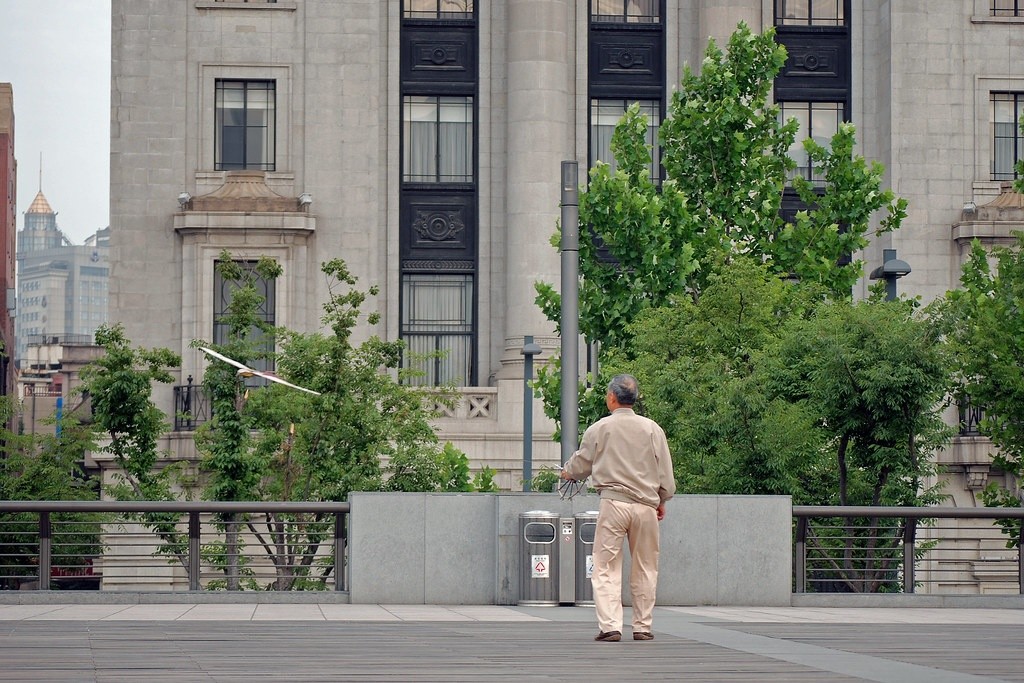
[595,631,622,640]
[632,632,655,640]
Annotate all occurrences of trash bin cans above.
[518,510,600,608]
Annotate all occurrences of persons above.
[562,374,675,642]
